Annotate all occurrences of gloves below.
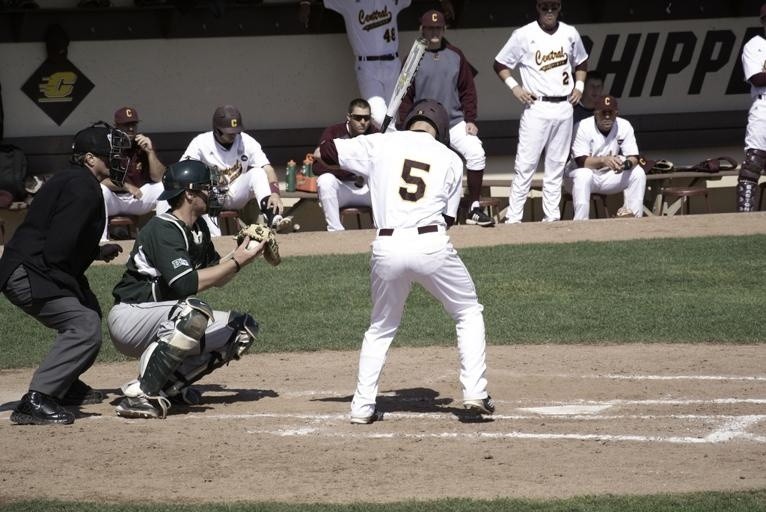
[94,242,124,264]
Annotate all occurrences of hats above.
[592,93,619,115]
[212,103,244,137]
[114,106,143,126]
[422,11,447,30]
[635,151,658,174]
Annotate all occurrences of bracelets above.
[575,80,584,93]
[232,257,241,273]
[270,182,281,195]
[299,1,310,5]
[624,160,633,170]
[504,76,519,90]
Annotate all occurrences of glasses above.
[349,113,372,122]
[538,5,561,12]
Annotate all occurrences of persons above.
[493,0,589,223]
[312,98,381,231]
[312,100,495,423]
[1,122,132,426]
[179,107,291,239]
[573,70,604,126]
[398,10,496,227]
[736,7,766,212]
[297,0,456,132]
[96,108,173,243]
[562,95,647,220]
[109,160,275,418]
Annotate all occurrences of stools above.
[336,203,376,232]
[557,189,613,223]
[453,196,503,230]
[102,211,141,242]
[212,207,242,241]
[651,183,713,220]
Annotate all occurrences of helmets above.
[68,122,132,189]
[156,160,227,217]
[399,98,451,146]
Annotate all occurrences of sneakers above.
[10,390,75,425]
[258,194,286,232]
[462,393,496,414]
[464,206,495,227]
[114,395,165,419]
[165,385,206,407]
[59,382,105,406]
[347,404,386,425]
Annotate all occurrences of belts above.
[358,52,399,62]
[531,95,570,102]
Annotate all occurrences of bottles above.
[300,153,314,177]
[285,159,298,191]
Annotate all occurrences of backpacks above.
[0,143,30,201]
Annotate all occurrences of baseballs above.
[294,224,301,230]
[247,240,260,250]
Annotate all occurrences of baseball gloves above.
[640,156,673,173]
[236,223,281,266]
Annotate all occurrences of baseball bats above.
[354,36,431,188]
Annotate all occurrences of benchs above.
[457,162,766,222]
